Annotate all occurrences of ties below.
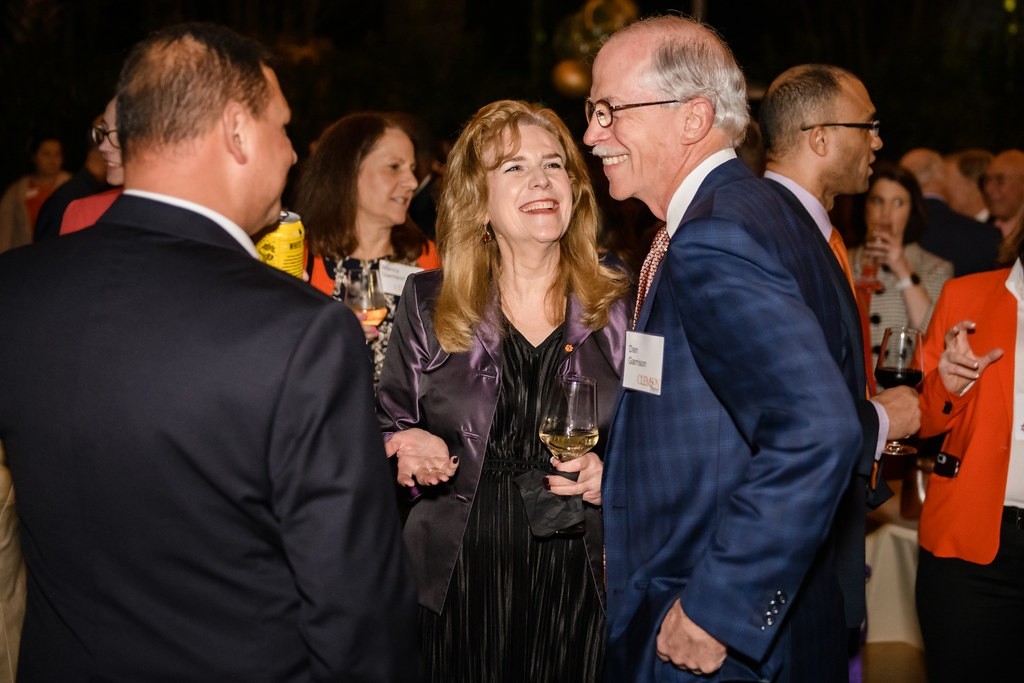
[829,225,874,398]
[632,225,671,331]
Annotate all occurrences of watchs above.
[898,273,920,288]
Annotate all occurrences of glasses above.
[93,125,121,149]
[585,100,693,128]
[802,121,880,137]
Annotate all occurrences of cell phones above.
[933,452,961,478]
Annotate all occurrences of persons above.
[912,207,1024,683]
[377,99,637,683]
[901,149,1024,273]
[0,96,124,250]
[0,23,427,683]
[584,15,862,683]
[299,112,442,404]
[846,166,955,646]
[760,64,922,683]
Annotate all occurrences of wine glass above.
[874,327,923,457]
[863,227,892,295]
[538,374,599,534]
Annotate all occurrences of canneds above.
[252,209,305,282]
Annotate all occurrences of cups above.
[342,269,388,344]
